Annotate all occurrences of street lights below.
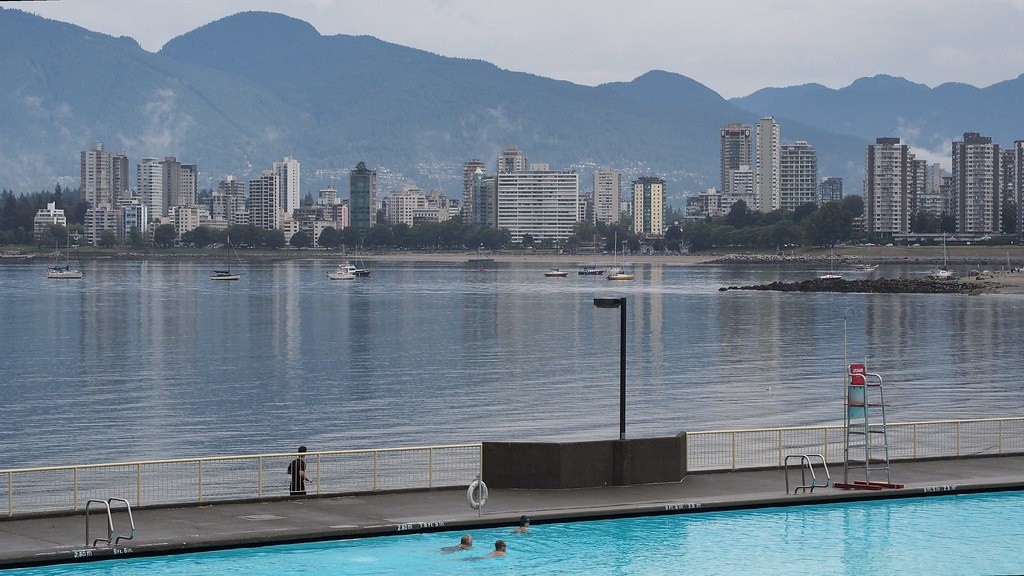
[593,297,629,441]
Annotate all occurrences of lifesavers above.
[467,480,489,509]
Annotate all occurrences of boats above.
[855,264,879,271]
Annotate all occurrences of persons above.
[492,540,506,556]
[519,515,530,532]
[459,534,472,548]
[288,446,313,494]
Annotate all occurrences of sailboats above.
[207,234,249,280]
[816,249,843,280]
[47,221,86,279]
[325,239,371,281]
[926,232,955,280]
[542,240,569,278]
[577,234,605,276]
[605,245,625,275]
[605,231,635,281]
